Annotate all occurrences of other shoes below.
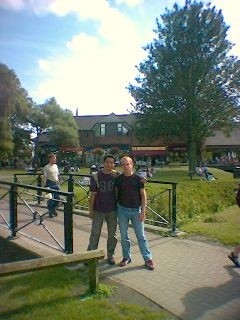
[144,260,155,270]
[48,207,57,218]
[107,257,115,265]
[119,259,131,266]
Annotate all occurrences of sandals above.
[228,252,240,268]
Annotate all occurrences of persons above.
[42,153,60,218]
[195,164,206,182]
[137,166,156,180]
[114,156,156,270]
[227,244,240,267]
[87,154,147,265]
